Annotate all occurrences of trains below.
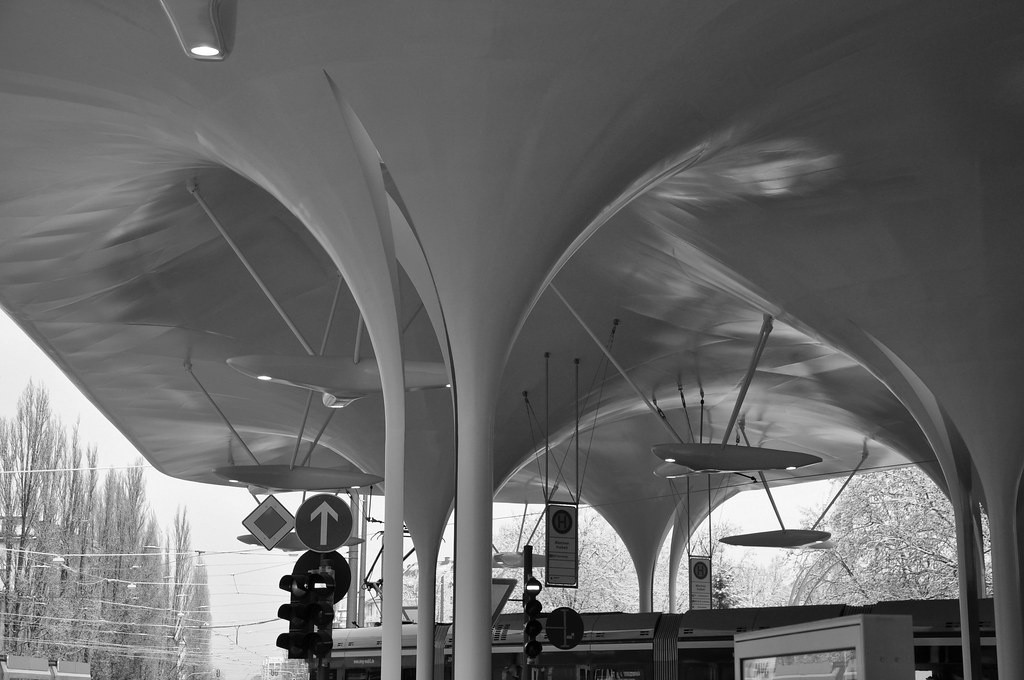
[301,598,1003,680]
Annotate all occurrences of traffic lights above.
[523,577,543,657]
[304,566,334,660]
[275,568,309,659]
